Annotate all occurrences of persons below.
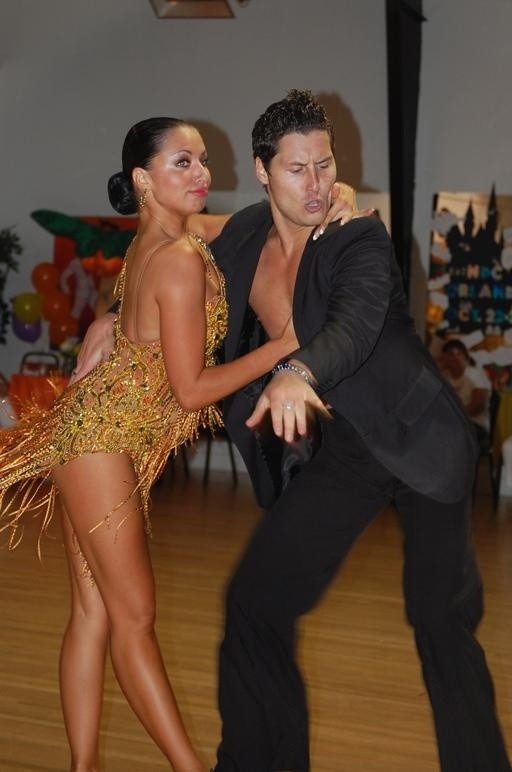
[0,119,376,770]
[439,335,494,450]
[66,86,512,771]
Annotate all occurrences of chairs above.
[181,421,241,490]
[472,388,502,515]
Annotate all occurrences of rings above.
[281,402,295,412]
[71,370,77,376]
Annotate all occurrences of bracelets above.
[272,361,312,385]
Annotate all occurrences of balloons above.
[10,262,75,343]
